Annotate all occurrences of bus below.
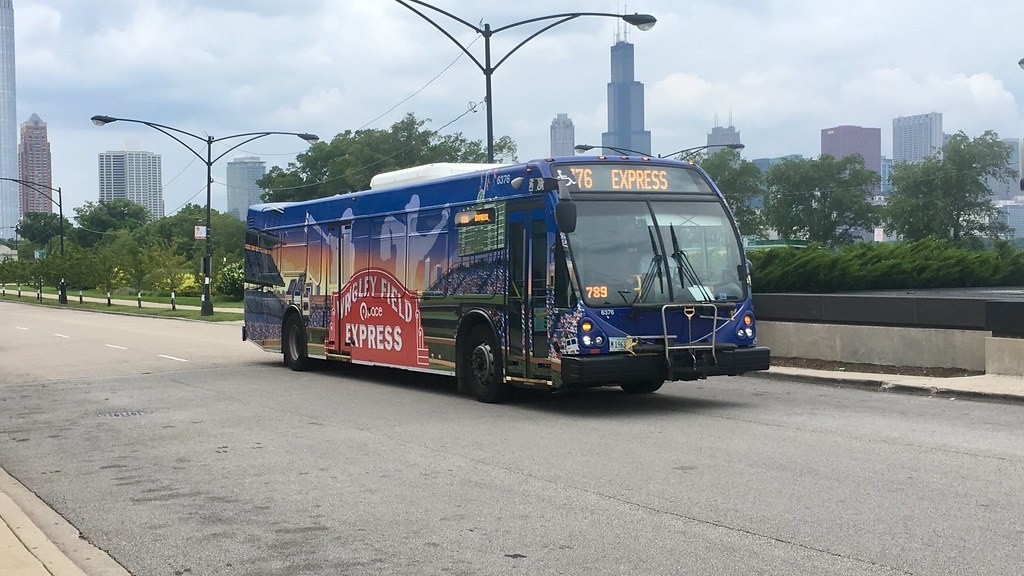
[242,155,770,404]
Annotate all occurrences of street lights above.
[396,0,657,164]
[90,115,319,315]
